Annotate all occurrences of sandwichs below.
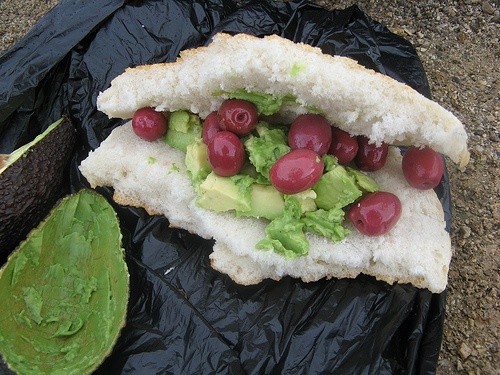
[77,32,469,295]
